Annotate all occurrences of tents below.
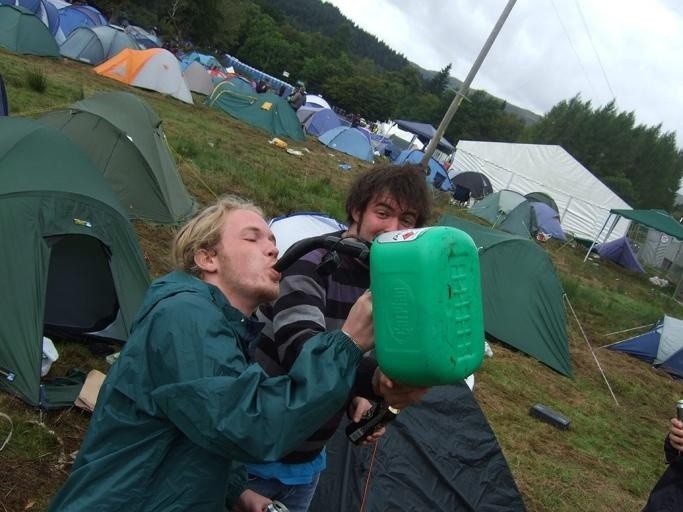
[608,314,681,379]
[0,0,683,284]
[3,105,156,403]
[440,212,577,375]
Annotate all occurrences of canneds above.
[676,400,683,421]
[345,401,401,446]
[262,501,290,512]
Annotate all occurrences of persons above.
[51,190,376,510]
[634,389,682,509]
[216,158,431,509]
